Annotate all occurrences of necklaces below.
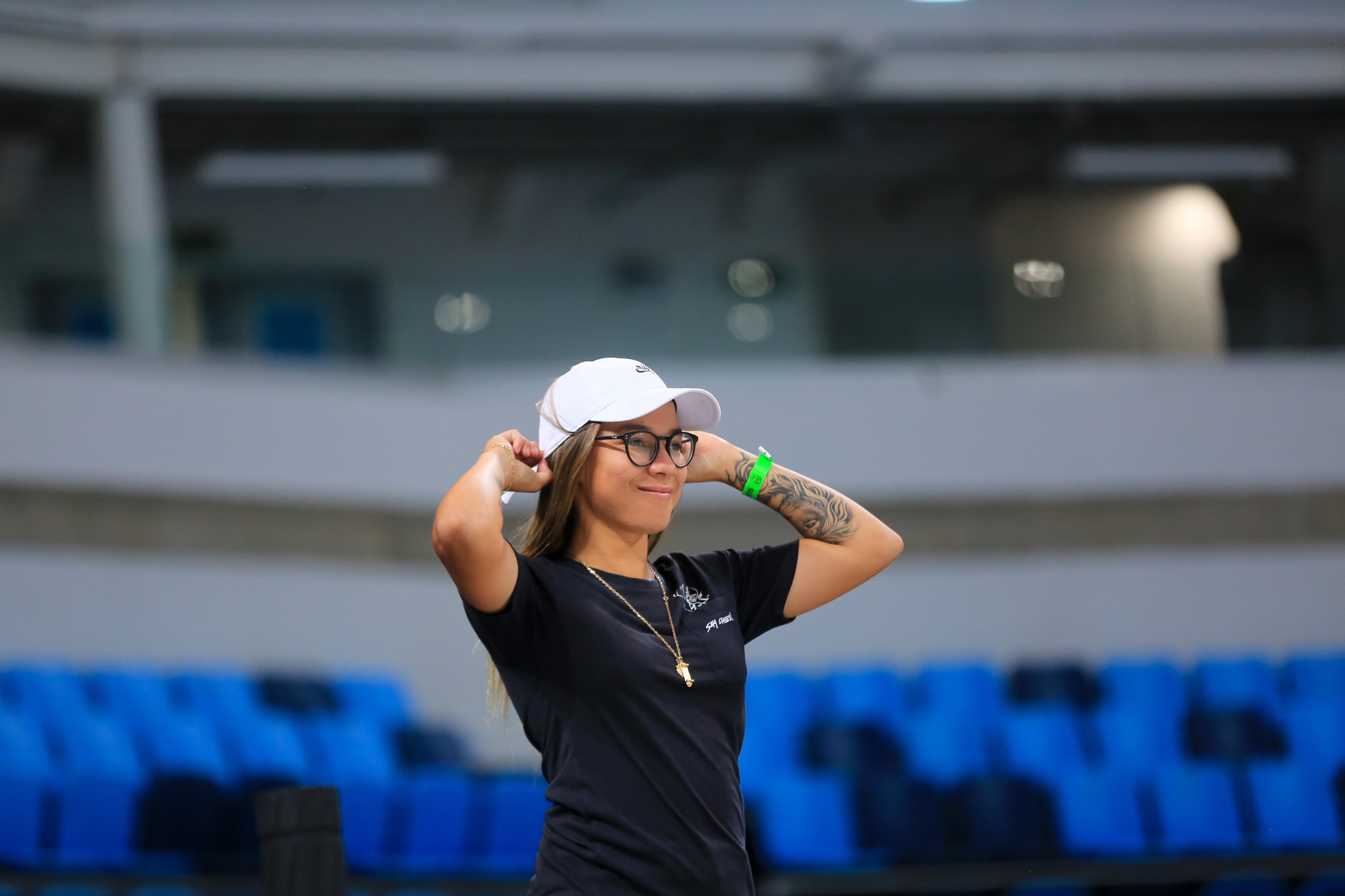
[560,550,695,690]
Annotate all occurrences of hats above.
[528,357,721,467]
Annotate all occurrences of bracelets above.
[741,444,773,500]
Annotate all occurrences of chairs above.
[0,639,1342,892]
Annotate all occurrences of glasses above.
[592,423,698,471]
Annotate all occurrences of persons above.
[432,355,906,896]
